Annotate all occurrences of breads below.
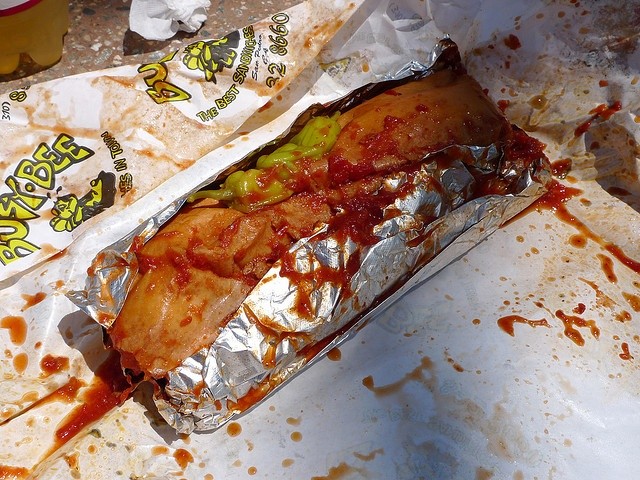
[102,57,544,379]
